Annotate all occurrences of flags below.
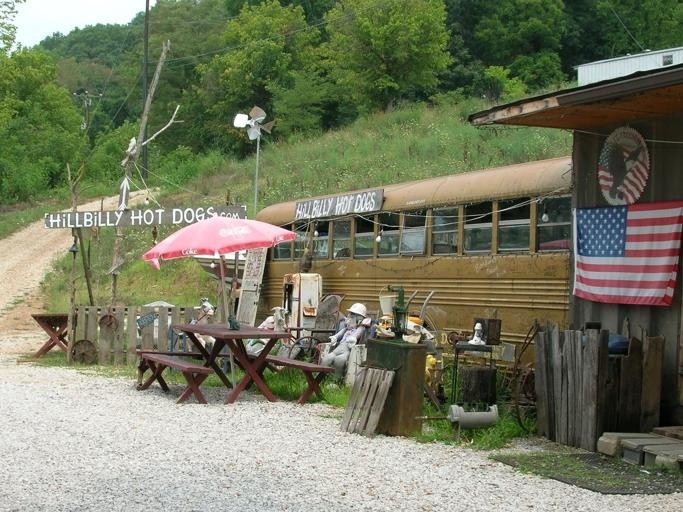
[572,201,683,308]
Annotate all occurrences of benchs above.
[135,349,266,390]
[139,353,214,404]
[245,356,336,405]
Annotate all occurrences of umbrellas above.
[143,212,298,390]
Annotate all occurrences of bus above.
[239,158,574,371]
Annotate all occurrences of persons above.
[306,303,368,391]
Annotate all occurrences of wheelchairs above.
[282,292,348,365]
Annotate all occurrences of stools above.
[451,341,492,411]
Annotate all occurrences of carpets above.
[487,453,683,494]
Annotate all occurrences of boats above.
[192,252,246,291]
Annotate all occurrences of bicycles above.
[513,360,535,436]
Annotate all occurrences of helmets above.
[346,303,368,318]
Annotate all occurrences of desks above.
[31,313,77,358]
[181,323,292,405]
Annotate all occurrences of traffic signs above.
[43,204,246,229]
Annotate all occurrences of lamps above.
[542,201,549,222]
[375,214,381,242]
[314,221,319,237]
